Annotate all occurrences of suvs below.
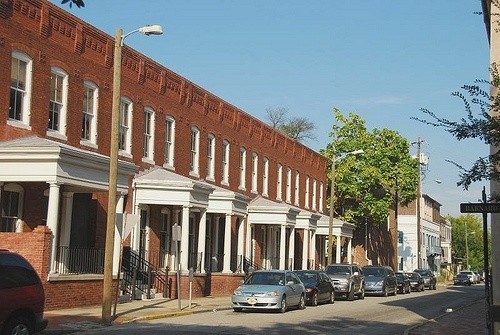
[362,265,398,296]
[322,264,366,301]
[414,269,437,290]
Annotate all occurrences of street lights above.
[466,232,476,270]
[327,149,365,265]
[100,25,164,325]
[417,179,442,269]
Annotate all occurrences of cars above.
[405,272,425,292]
[454,273,471,286]
[0,248,48,335]
[459,271,480,284]
[293,270,336,306]
[231,269,306,313]
[395,272,411,294]
